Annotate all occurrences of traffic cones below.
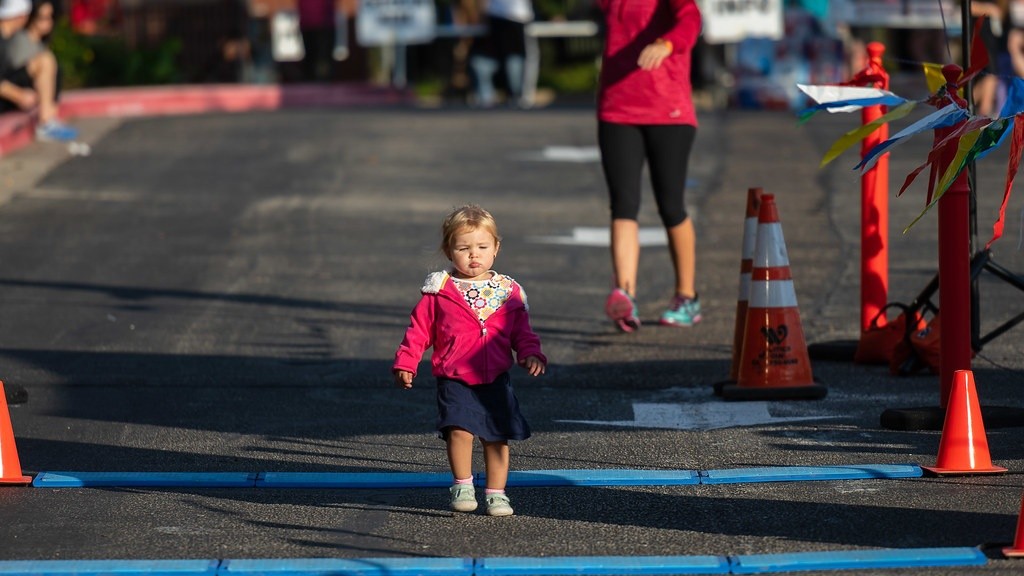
[1002,496,1024,558]
[920,371,1010,475]
[0,381,33,483]
[713,186,830,403]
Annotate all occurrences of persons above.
[0,0,76,148]
[596,0,701,333]
[970,0,1024,117]
[392,204,546,517]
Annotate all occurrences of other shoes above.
[34,122,79,143]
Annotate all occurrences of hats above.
[0,0,33,19]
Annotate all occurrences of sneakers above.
[449,484,478,511]
[485,492,514,516]
[659,290,703,327]
[606,289,641,334]
